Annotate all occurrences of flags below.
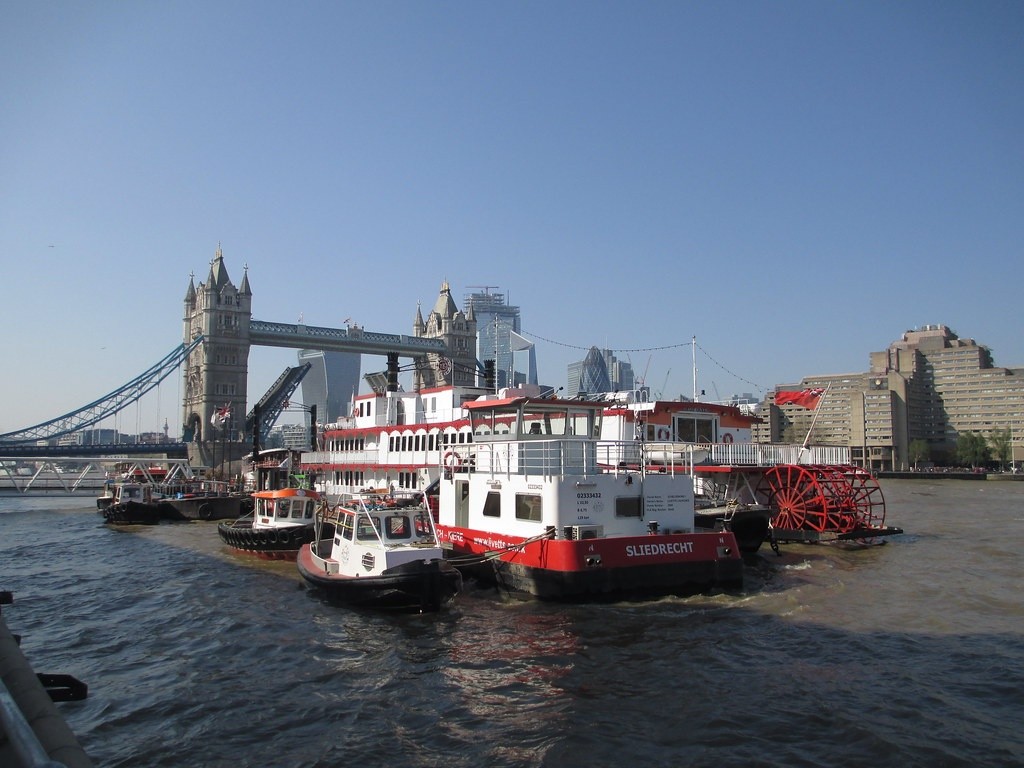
[775,389,824,412]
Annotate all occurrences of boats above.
[690,479,781,563]
[298,484,465,611]
[102,481,159,523]
[477,332,904,549]
[216,485,339,563]
[93,458,243,522]
[237,447,309,501]
[301,313,744,599]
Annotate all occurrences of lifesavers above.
[658,428,670,440]
[722,433,734,444]
[444,451,462,471]
[354,407,359,417]
